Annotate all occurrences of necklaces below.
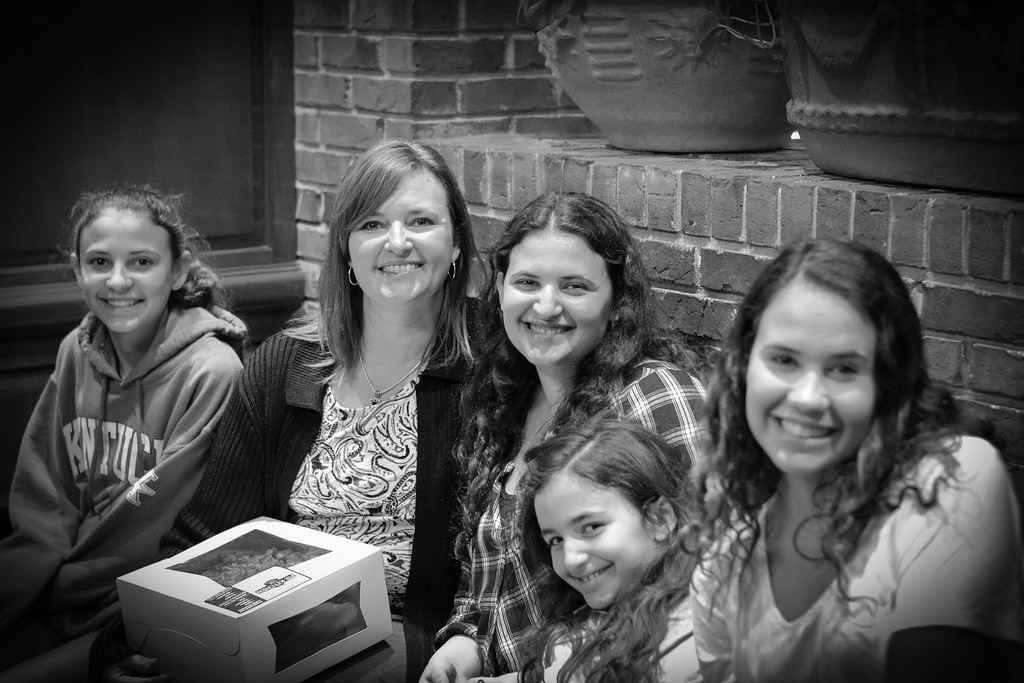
[358,340,436,405]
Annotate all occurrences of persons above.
[88,139,503,683]
[418,190,706,683]
[689,236,1024,683]
[0,185,247,683]
[515,424,706,683]
